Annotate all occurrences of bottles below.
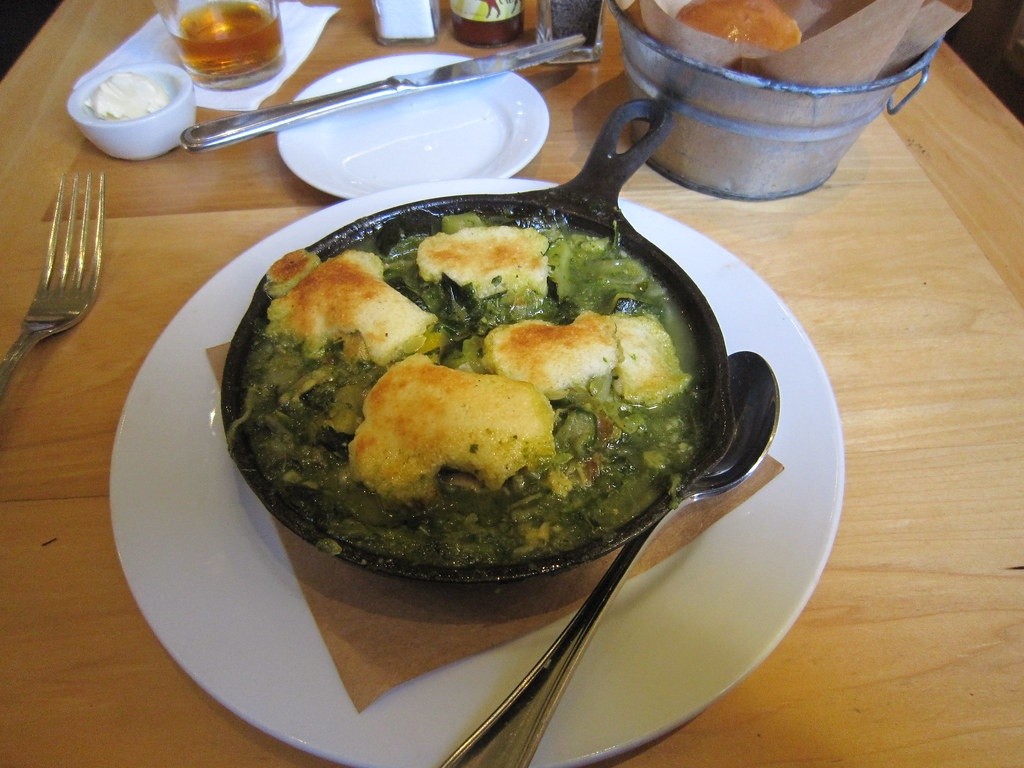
[449,0,525,48]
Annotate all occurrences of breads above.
[675,0,802,52]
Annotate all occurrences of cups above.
[369,0,442,47]
[149,0,287,92]
[536,0,605,64]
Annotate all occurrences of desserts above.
[83,71,172,120]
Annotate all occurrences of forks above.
[0,171,106,402]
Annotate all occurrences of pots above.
[220,99,741,586]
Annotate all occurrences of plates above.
[109,178,843,768]
[277,53,550,200]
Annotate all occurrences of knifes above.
[178,33,586,156]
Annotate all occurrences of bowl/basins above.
[66,61,197,162]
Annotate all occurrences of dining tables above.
[0,0,1024,768]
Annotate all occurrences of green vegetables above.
[227,212,705,568]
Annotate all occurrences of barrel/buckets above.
[605,0,946,202]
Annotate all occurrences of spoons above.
[434,351,782,768]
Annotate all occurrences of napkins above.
[72,0,341,112]
